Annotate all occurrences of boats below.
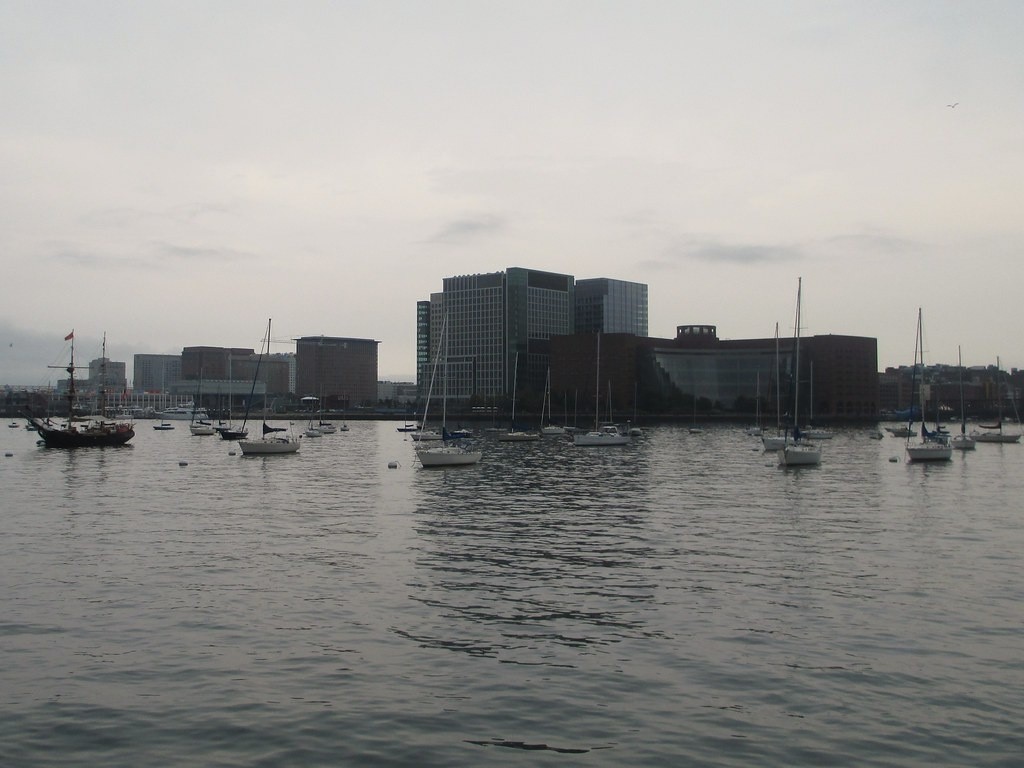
[8,421,20,428]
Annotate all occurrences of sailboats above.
[104,318,349,456]
[884,305,1024,460]
[20,327,136,445]
[397,331,643,446]
[745,275,836,467]
[688,368,704,434]
[414,305,482,467]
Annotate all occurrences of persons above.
[450,440,473,452]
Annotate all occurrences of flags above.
[64,332,73,341]
